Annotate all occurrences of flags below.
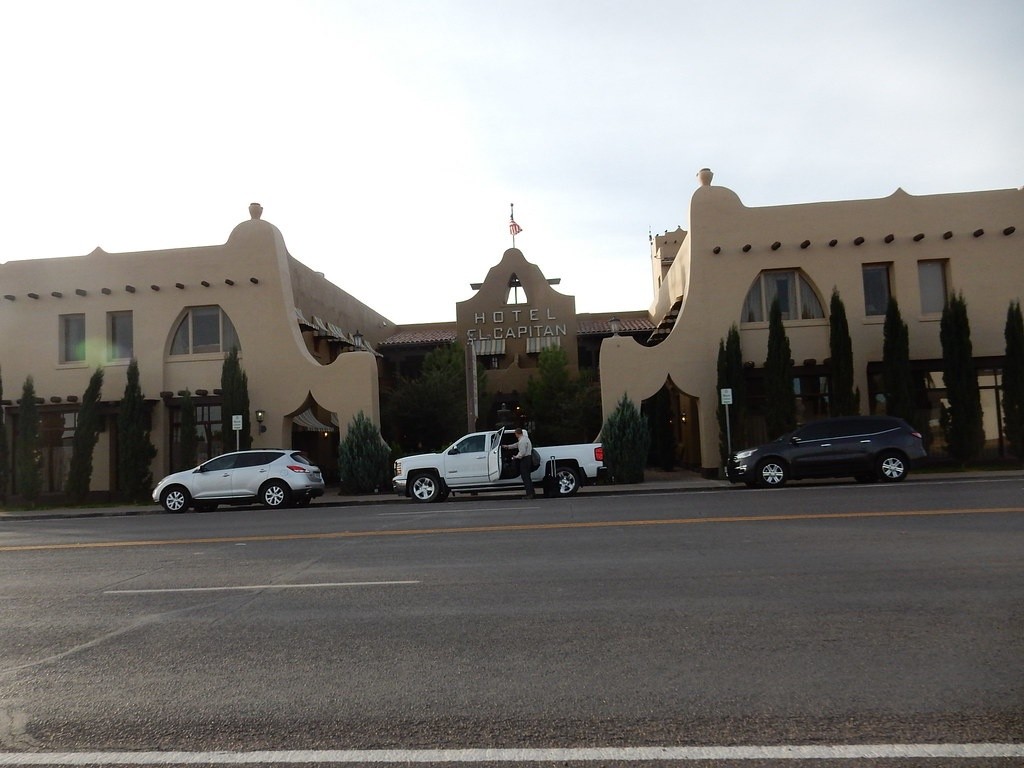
[509,214,522,235]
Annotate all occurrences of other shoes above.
[522,494,534,499]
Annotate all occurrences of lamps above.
[607,315,621,336]
[255,410,266,436]
[353,329,364,351]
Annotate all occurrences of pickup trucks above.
[392,424,609,503]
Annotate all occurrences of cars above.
[151,450,326,514]
[724,412,930,489]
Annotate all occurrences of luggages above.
[543,457,562,498]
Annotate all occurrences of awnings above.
[294,410,335,432]
[348,334,384,357]
[326,322,355,346]
[646,301,682,342]
[866,362,892,394]
[295,307,319,332]
[312,316,338,339]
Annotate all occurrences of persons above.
[502,428,535,499]
